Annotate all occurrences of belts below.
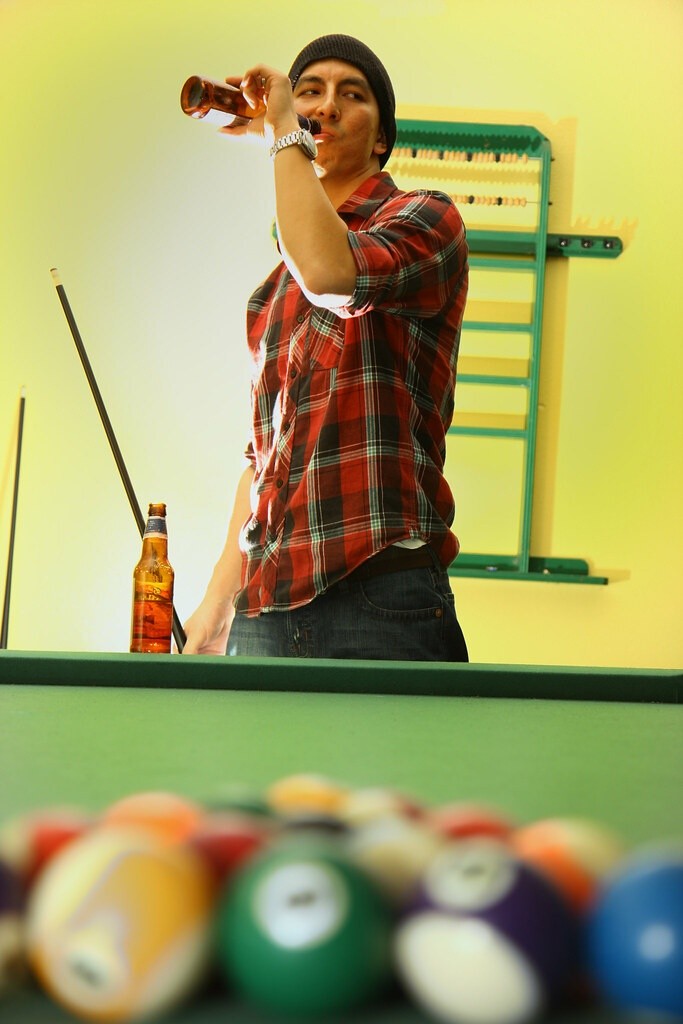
[342,551,435,584]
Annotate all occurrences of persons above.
[174,34,472,661]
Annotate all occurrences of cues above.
[50,266,188,654]
[1,385,28,649]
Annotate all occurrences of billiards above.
[1,774,683,1024]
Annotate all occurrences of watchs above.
[269,129,317,160]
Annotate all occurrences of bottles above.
[128,503,175,653]
[180,75,321,136]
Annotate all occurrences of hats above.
[288,34,396,170]
